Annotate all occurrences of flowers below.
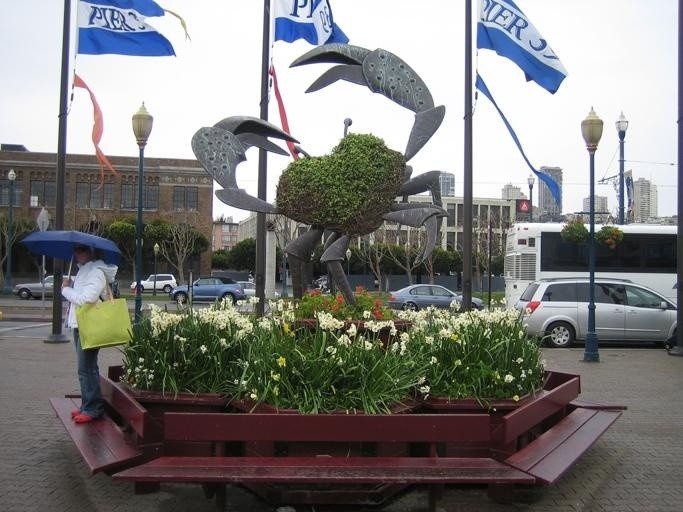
[111,284,553,416]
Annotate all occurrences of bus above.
[503,220,678,311]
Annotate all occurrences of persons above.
[60,242,119,424]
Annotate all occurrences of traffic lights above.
[514,198,529,215]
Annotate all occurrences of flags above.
[74,0,177,55]
[271,1,351,47]
[476,0,566,93]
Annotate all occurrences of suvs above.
[129,273,176,294]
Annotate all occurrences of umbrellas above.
[21,229,127,284]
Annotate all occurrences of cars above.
[386,284,485,314]
[234,280,279,298]
[169,275,243,306]
[12,274,74,301]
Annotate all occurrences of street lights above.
[153,242,159,295]
[526,172,536,221]
[132,231,144,329]
[579,104,605,362]
[609,106,630,223]
[131,98,155,326]
[344,247,352,278]
[2,168,17,293]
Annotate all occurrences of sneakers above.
[71,408,104,423]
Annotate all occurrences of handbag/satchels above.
[75,298,134,350]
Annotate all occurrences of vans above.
[510,275,678,348]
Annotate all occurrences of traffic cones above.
[133,287,137,297]
